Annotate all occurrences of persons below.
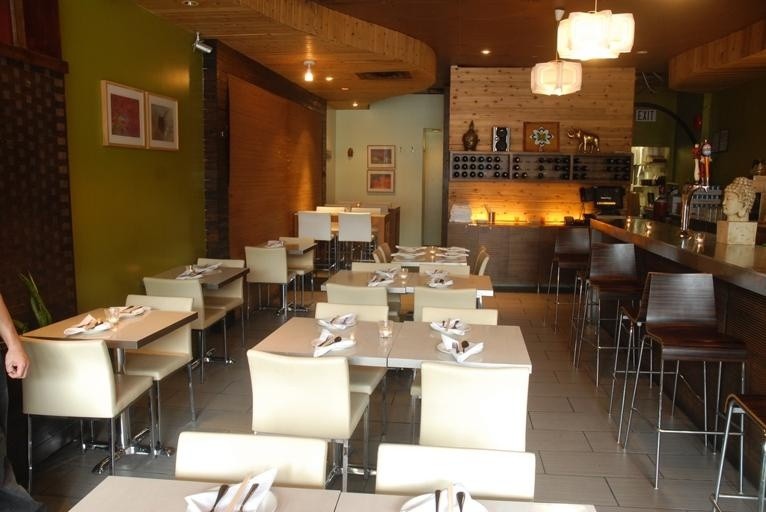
[722,176,756,222]
[0,296,29,380]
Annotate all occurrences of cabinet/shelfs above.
[449,151,634,184]
[447,223,508,283]
[509,227,557,284]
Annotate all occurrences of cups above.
[488,212,495,224]
[644,221,656,229]
[357,201,361,207]
[398,268,409,279]
[694,232,705,242]
[625,216,633,222]
[104,307,119,330]
[344,206,352,212]
[378,320,394,337]
[185,264,197,273]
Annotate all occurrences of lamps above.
[191,33,212,54]
[530,10,583,96]
[304,61,316,82]
[556,0,636,61]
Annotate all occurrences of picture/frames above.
[523,121,560,153]
[100,79,147,150]
[367,169,396,193]
[367,145,396,168]
[145,91,181,151]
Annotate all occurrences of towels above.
[197,263,222,272]
[176,274,207,279]
[386,271,395,278]
[438,247,470,252]
[435,253,469,256]
[185,467,279,511]
[110,307,151,315]
[390,252,427,256]
[313,329,354,357]
[63,314,116,336]
[426,271,448,277]
[368,279,394,286]
[401,483,472,511]
[441,334,484,363]
[432,320,464,336]
[429,278,453,286]
[268,241,284,247]
[395,245,427,250]
[318,312,355,330]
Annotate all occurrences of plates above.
[437,342,476,354]
[209,484,278,512]
[436,284,454,288]
[110,306,144,317]
[311,338,357,350]
[444,256,461,259]
[400,494,488,512]
[430,324,472,332]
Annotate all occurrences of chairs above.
[609,272,707,448]
[576,243,653,390]
[124,294,197,444]
[174,431,327,490]
[379,242,393,263]
[197,257,246,348]
[421,363,529,453]
[376,443,536,500]
[247,349,371,491]
[709,393,766,511]
[142,277,227,384]
[19,334,157,493]
[373,248,384,263]
[298,211,337,275]
[411,307,499,443]
[623,272,747,495]
[471,245,488,273]
[420,263,466,277]
[543,246,555,327]
[317,206,345,214]
[570,247,620,366]
[338,212,375,270]
[326,282,388,306]
[352,262,400,272]
[279,237,315,307]
[244,246,297,321]
[555,228,590,331]
[316,302,389,438]
[474,252,490,308]
[414,287,477,323]
[352,207,382,214]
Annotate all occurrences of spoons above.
[462,340,469,353]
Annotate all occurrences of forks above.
[452,342,459,354]
